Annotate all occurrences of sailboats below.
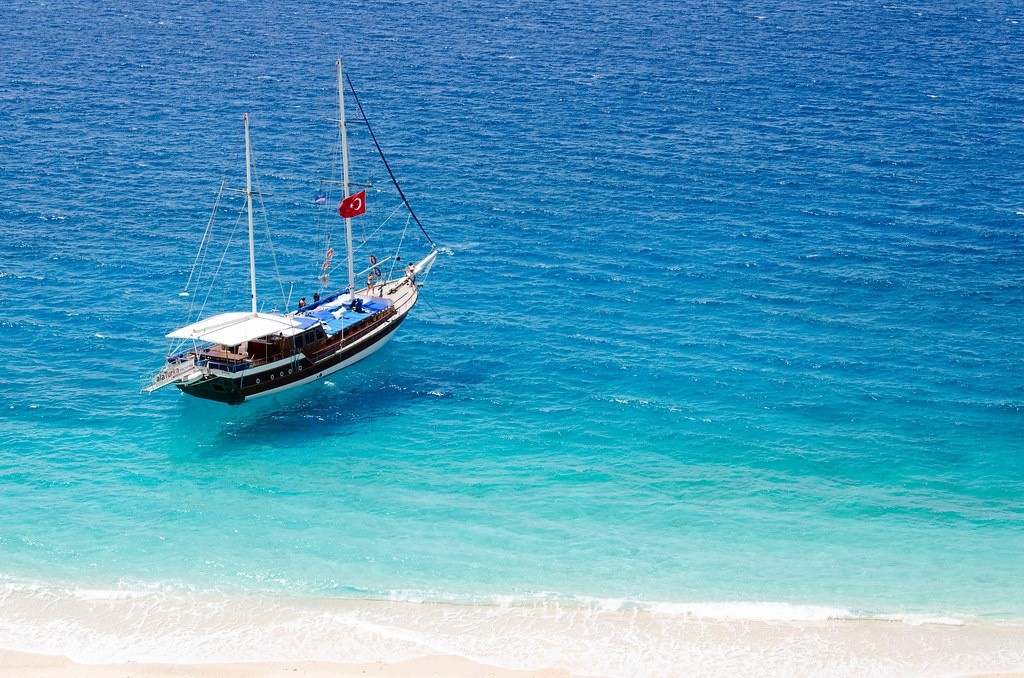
[141,59,443,406]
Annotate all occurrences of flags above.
[338,190,366,218]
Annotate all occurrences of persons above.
[313,293,320,302]
[366,272,377,296]
[408,263,415,286]
[298,297,306,317]
